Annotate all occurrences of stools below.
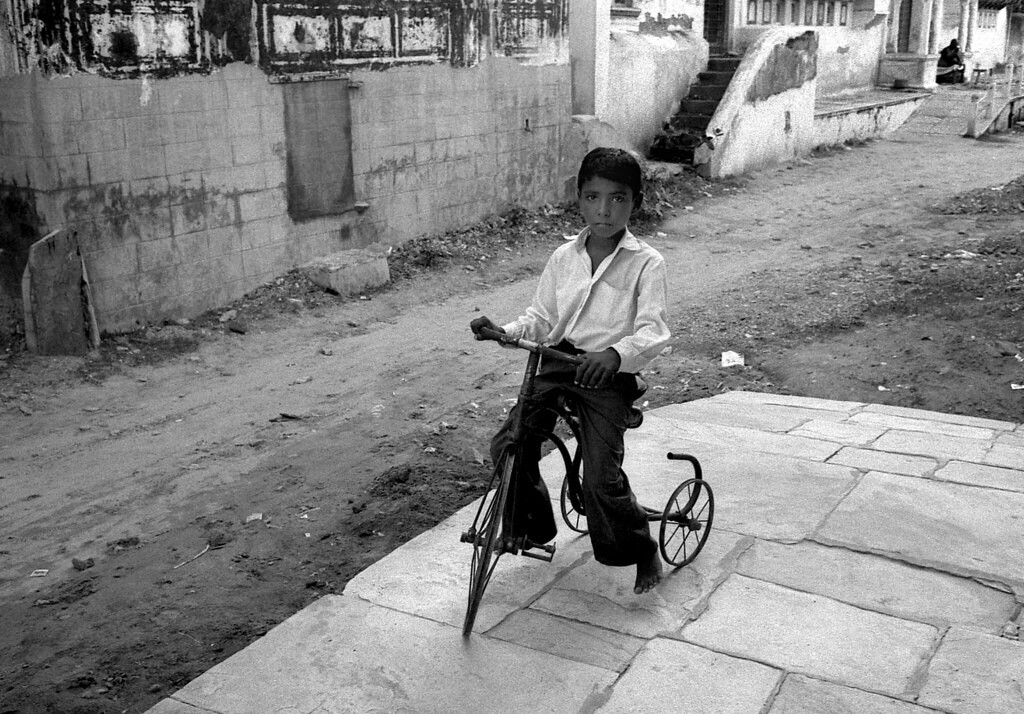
[970,69,992,90]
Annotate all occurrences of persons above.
[937,39,965,84]
[469,148,673,594]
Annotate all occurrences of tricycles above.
[459,325,716,636]
